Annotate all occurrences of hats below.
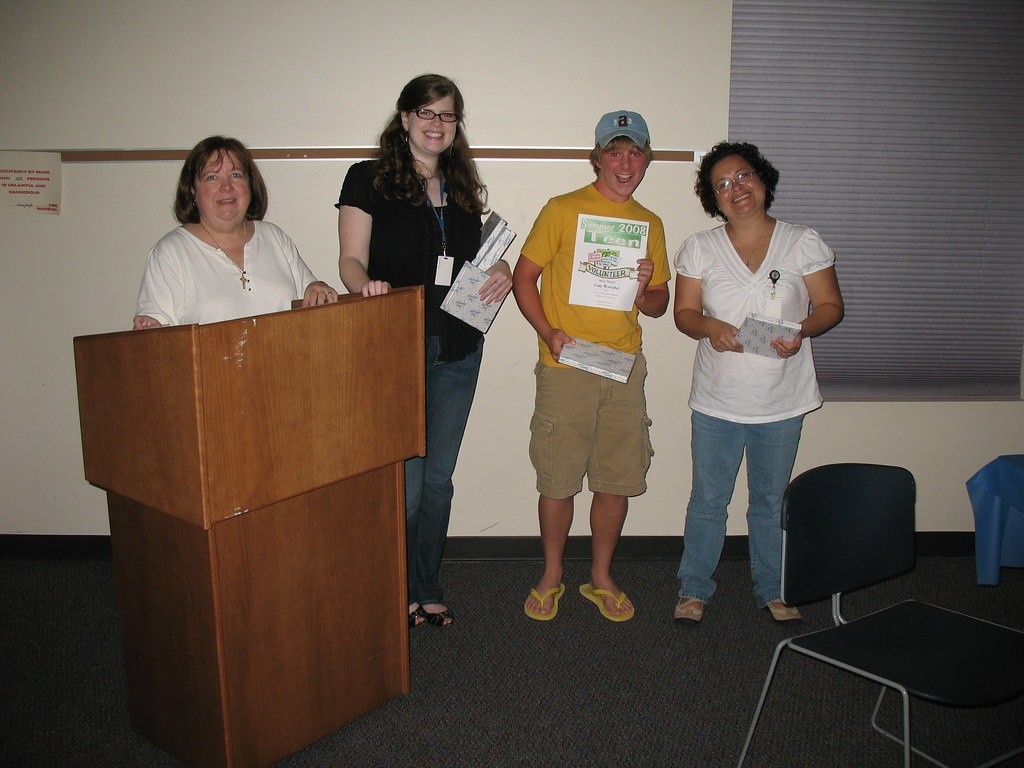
[594,110,650,151]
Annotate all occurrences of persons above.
[671,141,845,624]
[338,74,511,629]
[131,135,341,329]
[511,110,671,621]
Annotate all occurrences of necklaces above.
[198,219,251,294]
[726,217,769,271]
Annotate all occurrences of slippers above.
[579,583,635,622]
[523,582,565,620]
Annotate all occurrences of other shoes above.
[408,602,429,628]
[768,600,802,623]
[673,596,703,624]
[422,602,454,627]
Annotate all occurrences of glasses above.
[409,109,460,123]
[712,169,761,194]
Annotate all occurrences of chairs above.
[736,461,1024,768]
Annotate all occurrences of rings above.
[494,281,502,287]
[327,291,333,294]
